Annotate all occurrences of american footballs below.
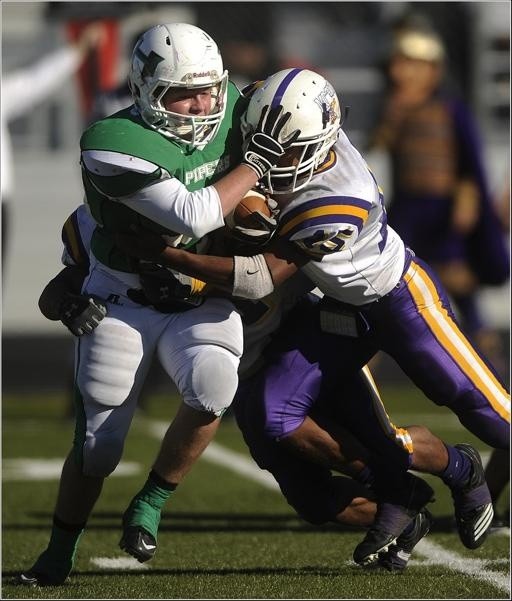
[231,191,270,225]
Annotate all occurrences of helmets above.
[127,22,229,149]
[238,68,342,195]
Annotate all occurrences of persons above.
[38,68,511,570]
[22,23,300,583]
[3,2,512,349]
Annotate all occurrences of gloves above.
[53,292,109,336]
[227,198,281,255]
[241,104,302,177]
[122,261,206,312]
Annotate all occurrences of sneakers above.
[354,442,494,573]
[119,478,176,563]
[19,547,73,589]
